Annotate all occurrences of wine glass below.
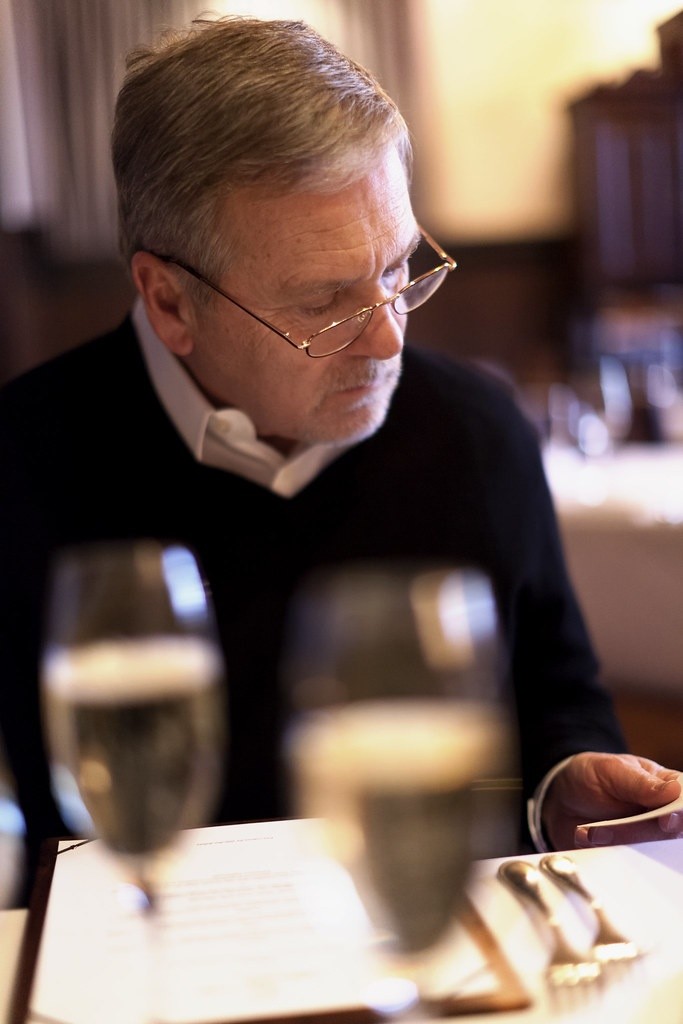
[271,565,515,1024]
[35,537,226,1024]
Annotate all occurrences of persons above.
[0,11,683,864]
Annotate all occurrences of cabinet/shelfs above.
[570,86,683,286]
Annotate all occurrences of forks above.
[538,855,650,968]
[496,858,601,991]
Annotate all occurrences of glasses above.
[128,220,457,358]
